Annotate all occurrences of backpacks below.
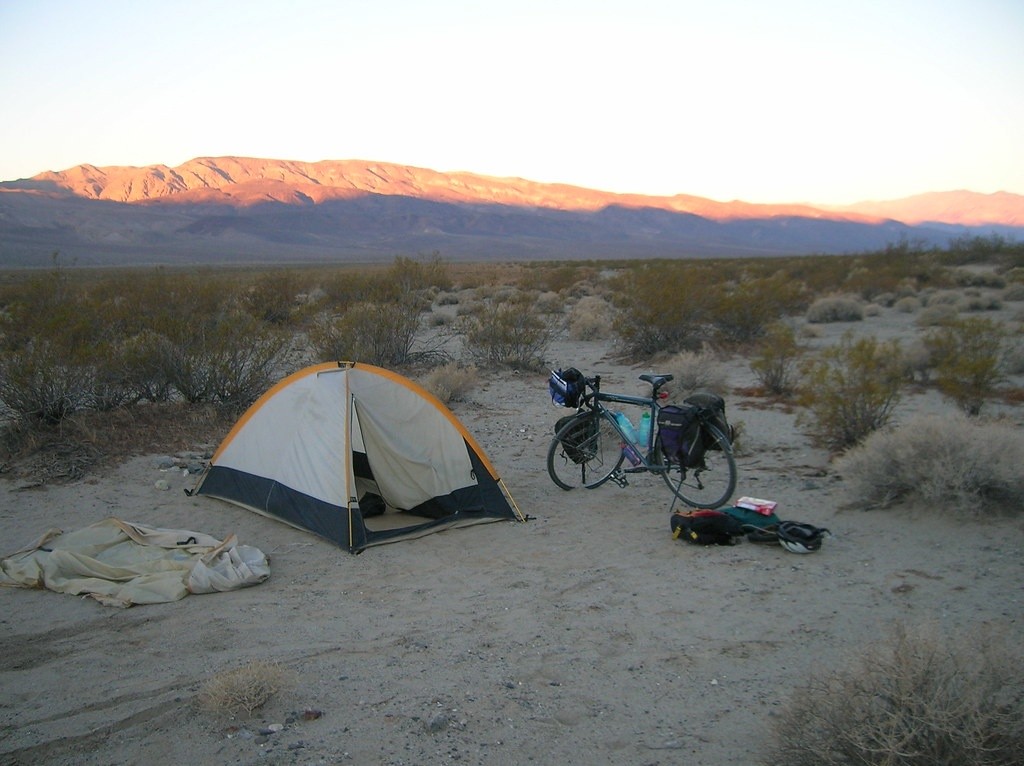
[670,508,745,547]
[657,404,706,470]
[554,399,599,464]
[684,393,734,450]
[722,502,782,538]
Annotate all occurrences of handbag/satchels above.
[549,366,586,408]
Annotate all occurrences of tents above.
[184,360,536,556]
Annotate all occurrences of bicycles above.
[546,367,738,514]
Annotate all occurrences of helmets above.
[779,520,832,554]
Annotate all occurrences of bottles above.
[621,445,641,467]
[616,413,638,443]
[639,412,650,447]
